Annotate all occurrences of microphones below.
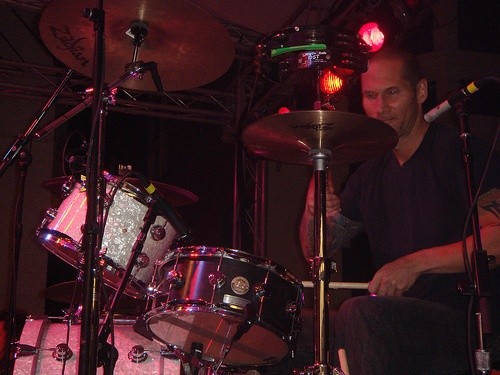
[423,80,482,123]
[135,172,193,241]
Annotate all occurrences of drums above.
[35,173,193,300]
[133,246,304,370]
[12,317,181,375]
[256,24,371,83]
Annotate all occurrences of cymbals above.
[46,280,146,315]
[240,110,399,166]
[39,0,235,92]
[41,175,199,208]
